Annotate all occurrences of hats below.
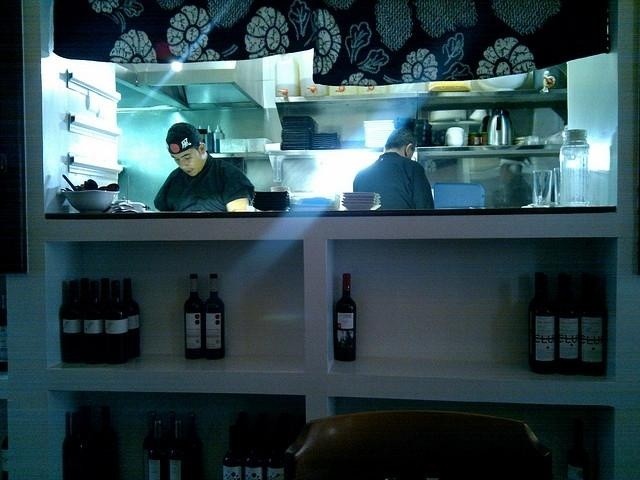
[167,123,201,154]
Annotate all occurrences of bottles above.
[59,276,142,365]
[143,412,197,480]
[203,273,226,359]
[219,411,294,480]
[197,124,224,153]
[558,127,589,204]
[65,408,106,480]
[530,272,607,375]
[185,274,206,360]
[333,272,357,362]
[607,124,617,205]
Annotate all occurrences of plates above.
[253,186,383,213]
[280,116,364,151]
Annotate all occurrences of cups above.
[532,169,553,205]
[555,169,563,204]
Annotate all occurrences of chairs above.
[277,409,556,479]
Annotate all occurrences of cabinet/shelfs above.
[267,86,568,184]
[43,205,638,479]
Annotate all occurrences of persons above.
[153,122,253,213]
[354,129,435,209]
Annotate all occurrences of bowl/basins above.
[479,73,529,90]
[61,190,119,213]
[412,118,434,147]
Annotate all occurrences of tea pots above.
[489,108,514,147]
